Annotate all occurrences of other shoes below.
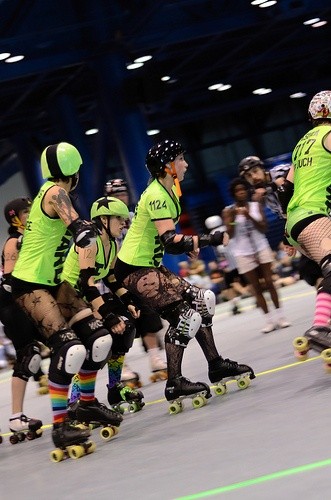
[277,319,289,327]
[263,323,276,333]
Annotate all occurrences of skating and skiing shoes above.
[106,384,145,414]
[120,365,142,389]
[208,359,255,395]
[72,397,122,441]
[150,358,169,383]
[50,420,95,462]
[292,325,331,373]
[164,377,211,415]
[9,414,43,445]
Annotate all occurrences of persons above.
[286,90,331,371]
[0,141,291,464]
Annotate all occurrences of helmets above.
[41,142,82,179]
[205,215,222,228]
[308,91,331,122]
[146,139,185,169]
[239,156,264,177]
[91,198,129,221]
[105,179,128,195]
[4,200,32,224]
